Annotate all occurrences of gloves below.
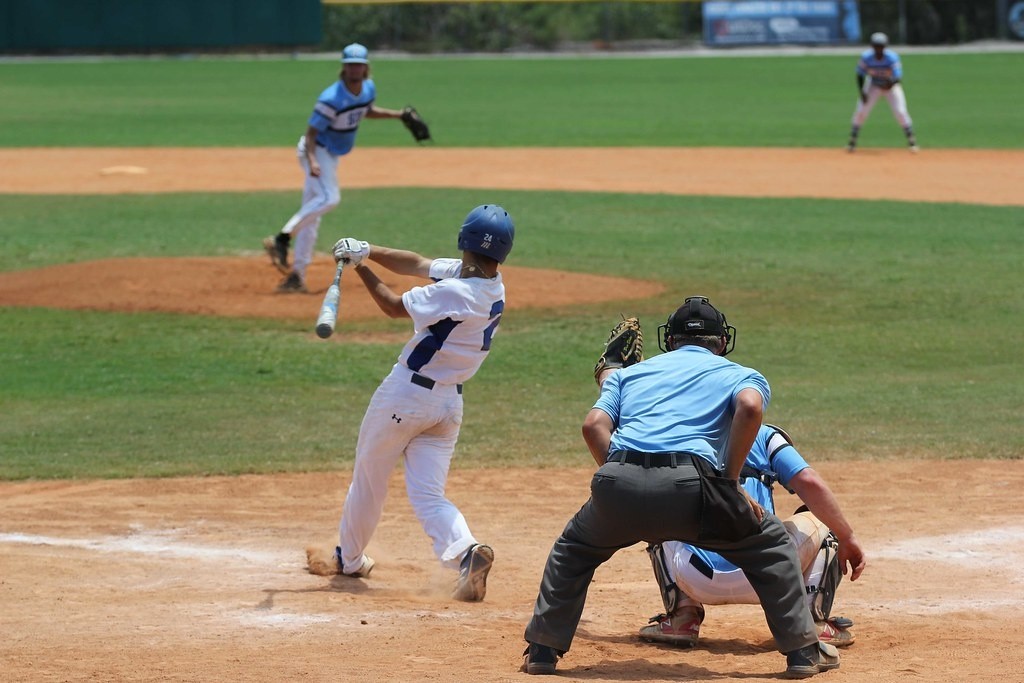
[332,238,370,266]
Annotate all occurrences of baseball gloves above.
[592,311,645,387]
[400,105,436,147]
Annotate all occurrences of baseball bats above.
[315,258,345,339]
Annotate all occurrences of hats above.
[667,299,723,335]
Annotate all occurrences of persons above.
[264,44,429,294]
[846,32,920,154]
[517,294,841,679]
[635,419,865,646]
[304,202,515,602]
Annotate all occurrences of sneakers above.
[786,642,841,679]
[639,606,700,646]
[264,232,291,273]
[452,543,494,602]
[814,619,855,645]
[525,642,558,674]
[283,269,305,291]
[336,546,375,577]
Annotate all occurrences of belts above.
[608,450,694,466]
[316,140,325,148]
[690,554,714,579]
[411,373,463,395]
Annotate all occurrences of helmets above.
[341,43,369,64]
[871,32,888,45]
[458,204,514,264]
